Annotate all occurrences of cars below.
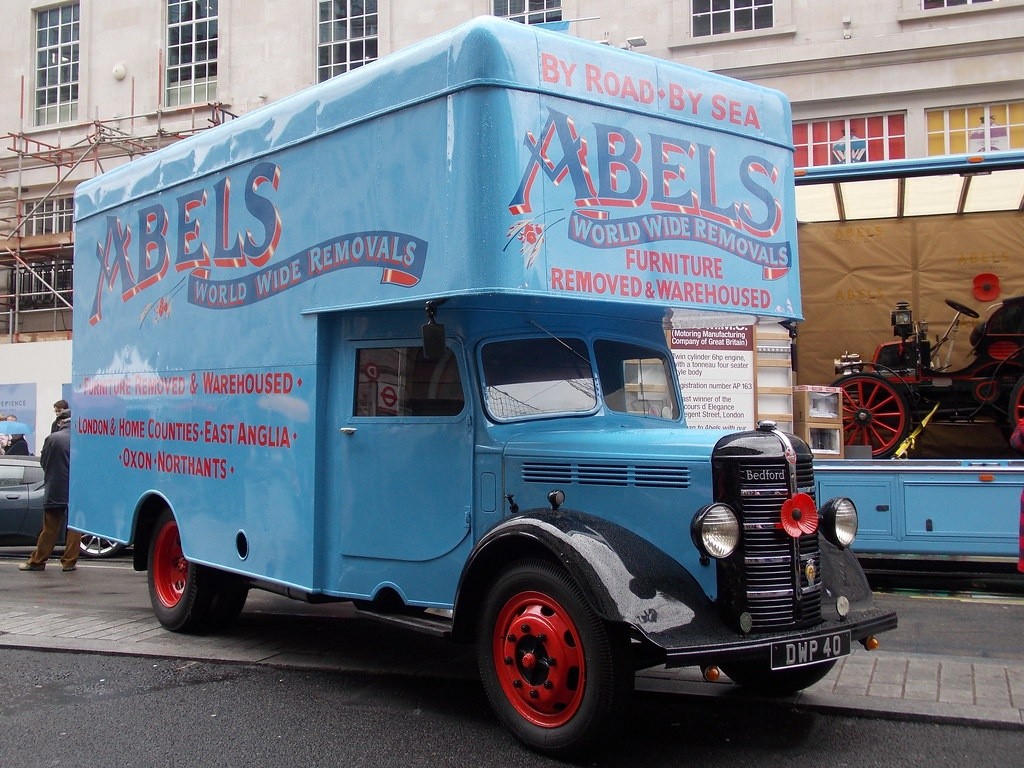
[826,295,1024,460]
[0,455,127,558]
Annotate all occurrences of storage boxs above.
[793,385,845,460]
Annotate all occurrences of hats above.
[56,409,71,422]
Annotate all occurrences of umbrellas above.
[0,421,32,447]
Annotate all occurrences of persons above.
[0,417,28,455]
[18,400,84,572]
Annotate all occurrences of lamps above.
[626,35,648,47]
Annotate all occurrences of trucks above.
[68,13,903,749]
[793,148,1024,578]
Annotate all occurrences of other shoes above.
[19,562,45,571]
[62,566,77,571]
